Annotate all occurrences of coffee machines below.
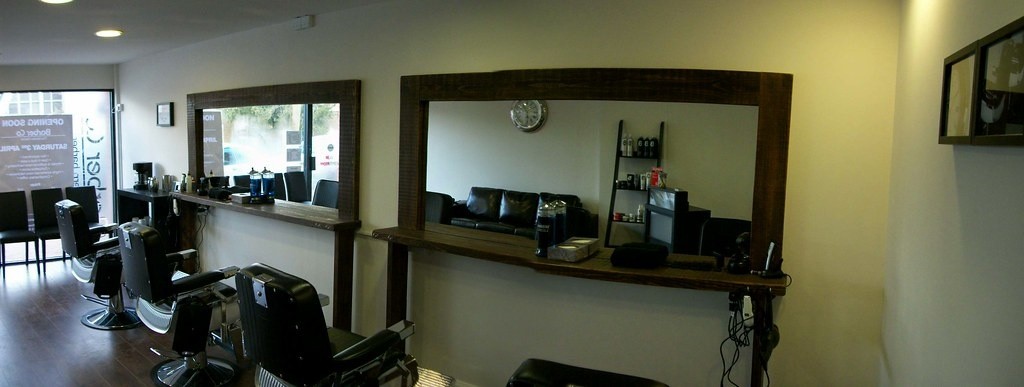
[132,163,152,191]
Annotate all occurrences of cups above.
[148,174,172,193]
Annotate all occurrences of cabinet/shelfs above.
[601,119,664,247]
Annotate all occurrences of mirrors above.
[186,79,361,230]
[396,67,793,295]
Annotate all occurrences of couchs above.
[449,186,599,249]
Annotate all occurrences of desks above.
[115,187,174,227]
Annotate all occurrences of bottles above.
[613,133,661,224]
[534,194,561,257]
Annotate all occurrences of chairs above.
[233,261,420,387]
[0,191,41,280]
[55,199,144,331]
[234,175,251,188]
[284,171,307,202]
[311,180,338,209]
[263,173,286,200]
[116,221,242,387]
[202,175,224,194]
[424,190,453,226]
[31,188,65,277]
[64,185,101,242]
[506,358,670,387]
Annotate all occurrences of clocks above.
[510,100,548,132]
